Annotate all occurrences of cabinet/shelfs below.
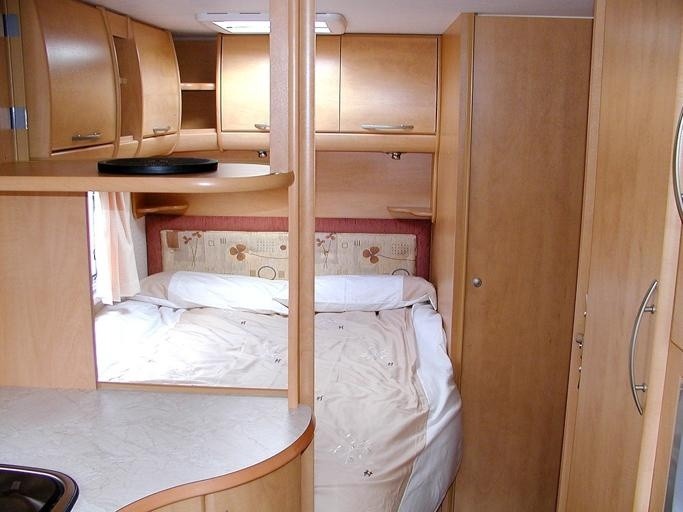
[14,2,181,163]
[432,12,594,512]
[174,30,444,157]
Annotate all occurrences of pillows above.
[312,269,437,312]
[133,270,289,317]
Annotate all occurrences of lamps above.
[197,10,347,40]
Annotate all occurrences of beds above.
[91,209,470,512]
